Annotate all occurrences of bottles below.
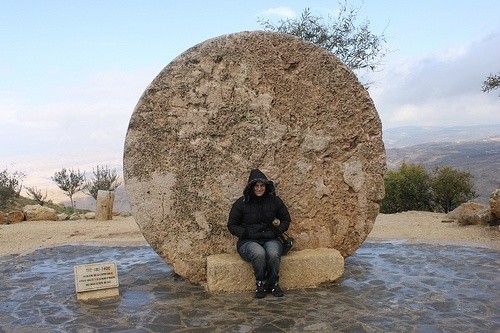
[272,218,280,228]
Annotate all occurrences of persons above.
[228,168,293,299]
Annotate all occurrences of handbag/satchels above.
[282,236,294,253]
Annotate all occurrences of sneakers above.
[254,285,267,298]
[268,284,283,297]
